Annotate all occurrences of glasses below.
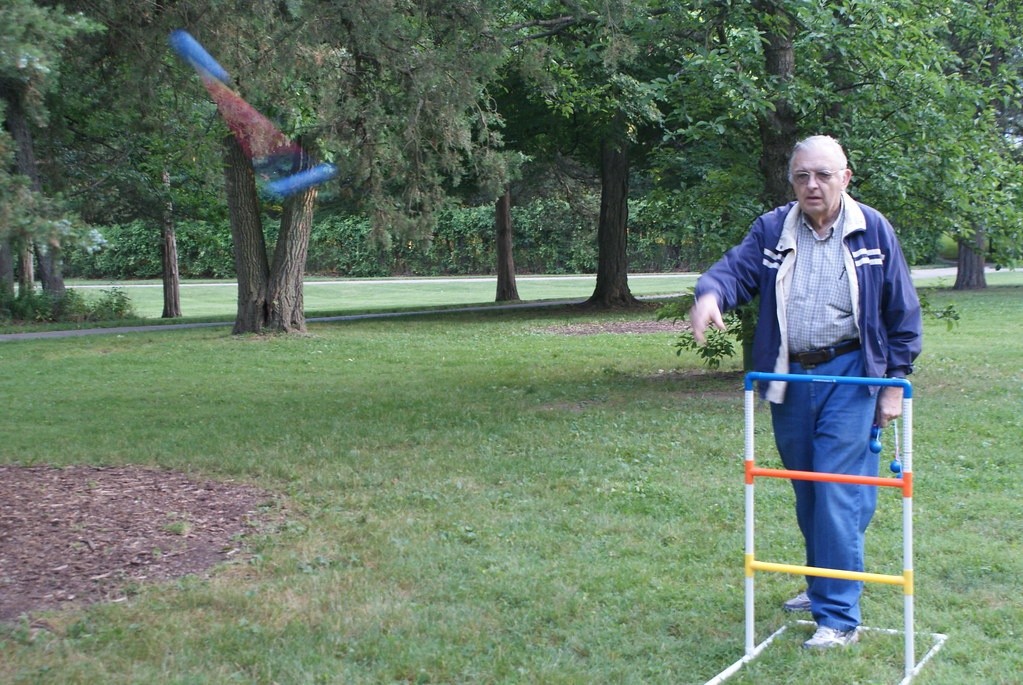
[790,169,840,184]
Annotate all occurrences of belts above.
[789,340,861,369]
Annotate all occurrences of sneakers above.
[783,591,811,611]
[802,624,860,649]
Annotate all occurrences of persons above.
[689,135,922,649]
